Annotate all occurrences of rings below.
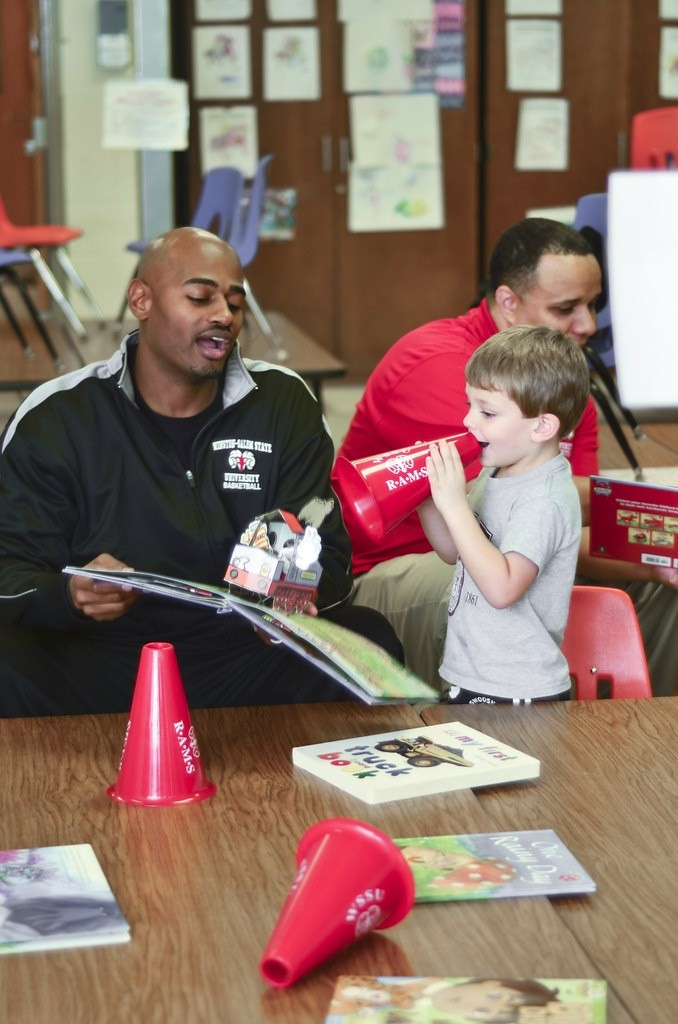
[91,579,100,593]
[270,638,283,644]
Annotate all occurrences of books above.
[385,825,596,906]
[0,844,132,955]
[589,474,678,570]
[290,720,541,803]
[62,510,442,708]
[324,972,609,1024]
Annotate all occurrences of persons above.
[411,325,582,704]
[0,225,407,725]
[327,216,678,705]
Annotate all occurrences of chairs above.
[1,696,678,1022]
[0,152,289,375]
